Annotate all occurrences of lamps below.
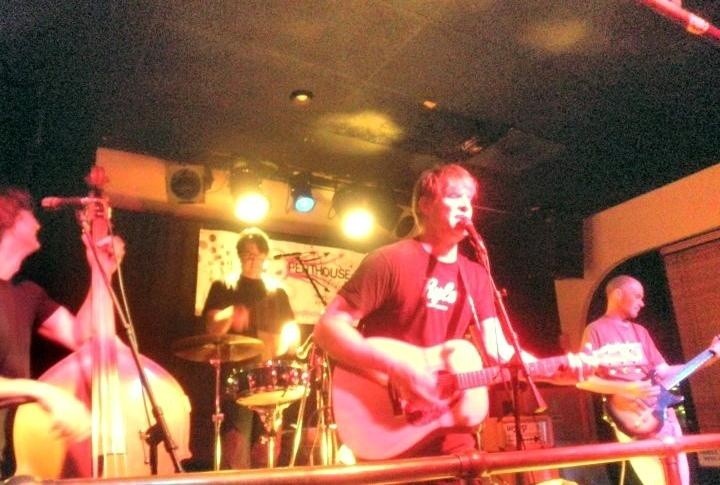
[226,155,377,241]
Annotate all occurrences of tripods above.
[287,361,344,468]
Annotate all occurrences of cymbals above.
[170,333,266,363]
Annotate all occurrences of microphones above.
[40,196,98,208]
[461,217,487,253]
[273,252,315,260]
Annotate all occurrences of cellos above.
[5,165,193,481]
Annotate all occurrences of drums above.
[305,342,332,393]
[224,359,312,410]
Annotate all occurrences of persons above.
[198,226,300,469]
[580,274,720,483]
[311,161,600,459]
[0,185,127,484]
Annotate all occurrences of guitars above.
[605,348,714,440]
[330,337,649,460]
